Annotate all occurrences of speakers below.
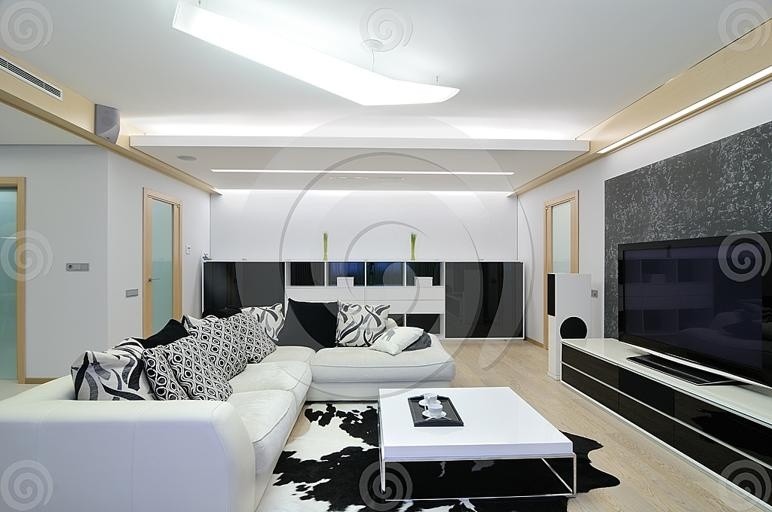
[547,272,592,382]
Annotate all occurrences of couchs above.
[0,324,456,512]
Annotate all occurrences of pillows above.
[188,319,248,380]
[335,301,390,347]
[181,314,218,332]
[159,335,233,402]
[131,318,190,348]
[221,314,277,363]
[142,344,190,400]
[71,338,157,401]
[403,331,432,352]
[240,303,285,341]
[368,326,423,355]
[274,298,339,352]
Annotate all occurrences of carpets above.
[255,401,620,512]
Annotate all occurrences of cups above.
[425,404,443,417]
[424,393,437,405]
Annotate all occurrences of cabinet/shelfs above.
[201,260,526,341]
[559,338,772,511]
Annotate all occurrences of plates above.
[418,399,441,407]
[408,393,464,427]
[422,410,447,418]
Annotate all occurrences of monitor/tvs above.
[619,235,768,389]
